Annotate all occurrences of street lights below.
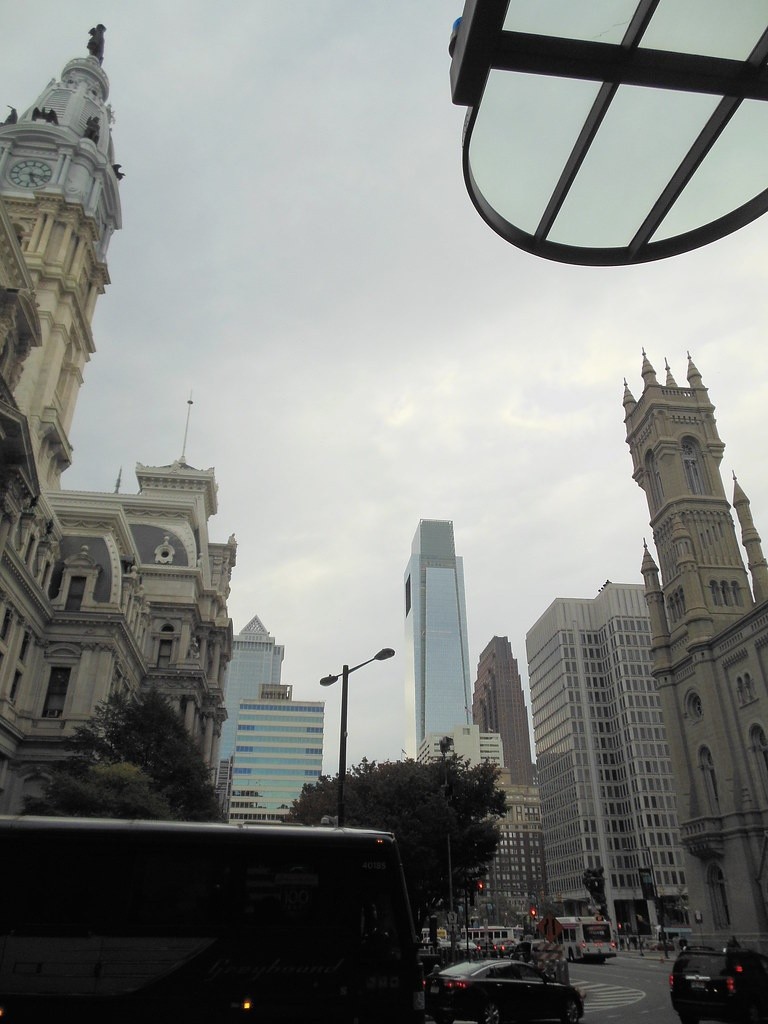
[623,845,670,960]
[319,644,396,831]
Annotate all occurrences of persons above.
[727,936,741,949]
[83,116,100,146]
[619,936,648,950]
[679,935,688,951]
[87,24,106,66]
[4,105,18,124]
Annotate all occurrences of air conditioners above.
[48,709,57,717]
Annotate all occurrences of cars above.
[422,936,451,949]
[423,957,586,1024]
[509,940,565,966]
[455,938,478,951]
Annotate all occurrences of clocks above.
[6,158,54,190]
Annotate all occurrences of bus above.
[535,915,618,965]
[0,811,456,1024]
[461,925,525,946]
[421,927,448,942]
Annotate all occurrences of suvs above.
[668,945,768,1023]
